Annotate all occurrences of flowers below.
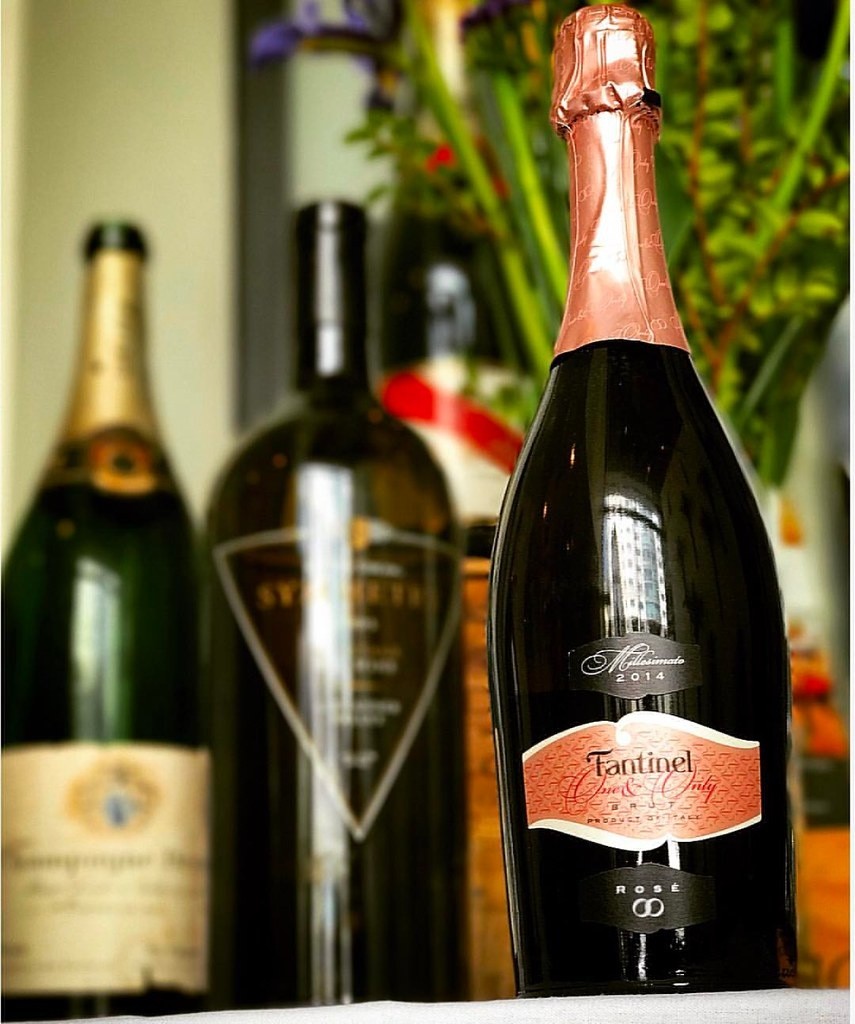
[242,1,850,510]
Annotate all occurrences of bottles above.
[791,674,850,989]
[0,219,205,1023]
[207,198,469,1011]
[485,5,799,999]
